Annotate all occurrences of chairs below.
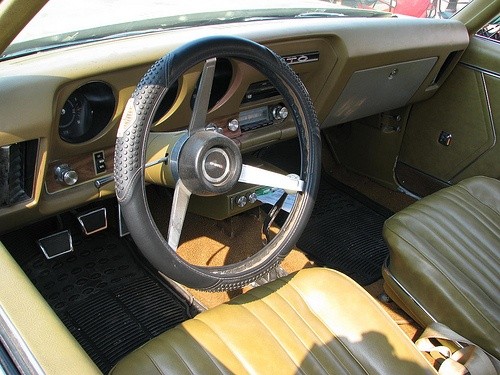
[106,267,442,375]
[382,176,500,372]
